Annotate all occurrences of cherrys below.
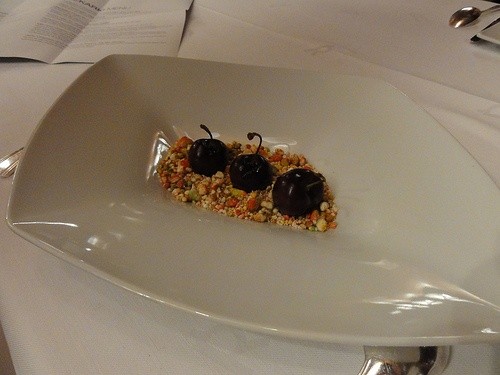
[273,168,326,217]
[188,125,229,176]
[229,132,273,192]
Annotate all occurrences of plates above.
[6,53,500,345]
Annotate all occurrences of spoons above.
[450,3,500,30]
